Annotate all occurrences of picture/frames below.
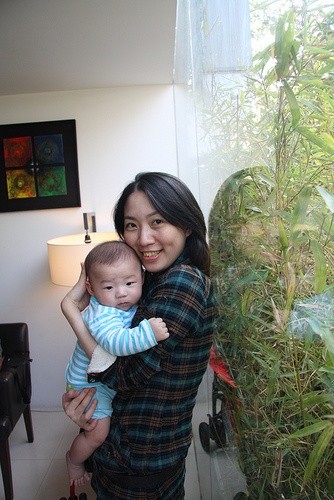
[0,117,83,215]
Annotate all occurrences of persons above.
[66,240,171,484]
[61,172,217,500]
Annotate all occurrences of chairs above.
[0,322,36,500]
